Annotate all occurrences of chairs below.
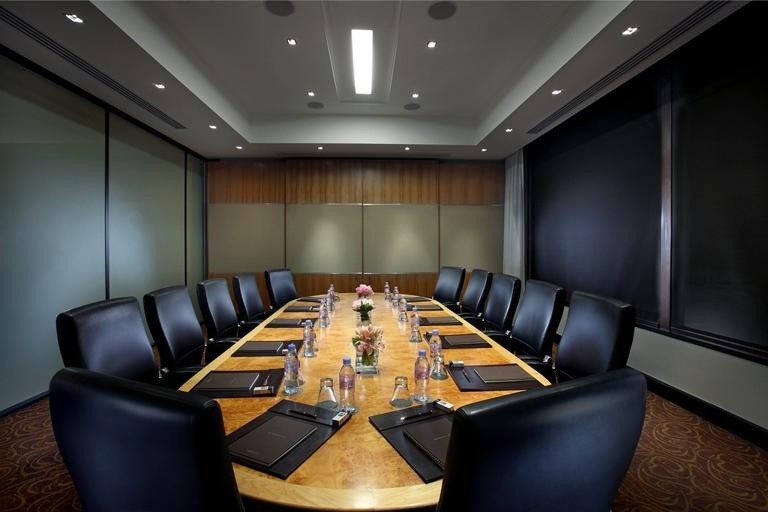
[48,267,297,510]
[432,265,647,511]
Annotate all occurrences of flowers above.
[356,284,374,298]
[352,323,386,365]
[352,298,376,313]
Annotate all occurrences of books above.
[403,419,453,471]
[286,305,311,312]
[405,295,427,302]
[228,415,318,467]
[444,334,488,347]
[194,372,260,394]
[301,295,320,302]
[426,316,461,325]
[270,319,302,326]
[237,341,285,353]
[474,365,535,383]
[413,304,441,310]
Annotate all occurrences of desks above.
[177,292,552,508]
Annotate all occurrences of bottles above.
[284,344,355,413]
[391,330,447,407]
[384,281,422,342]
[303,284,338,357]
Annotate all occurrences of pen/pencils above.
[288,409,317,419]
[263,375,271,385]
[405,410,433,420]
[462,369,472,383]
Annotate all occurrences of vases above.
[356,313,372,326]
[355,351,379,375]
